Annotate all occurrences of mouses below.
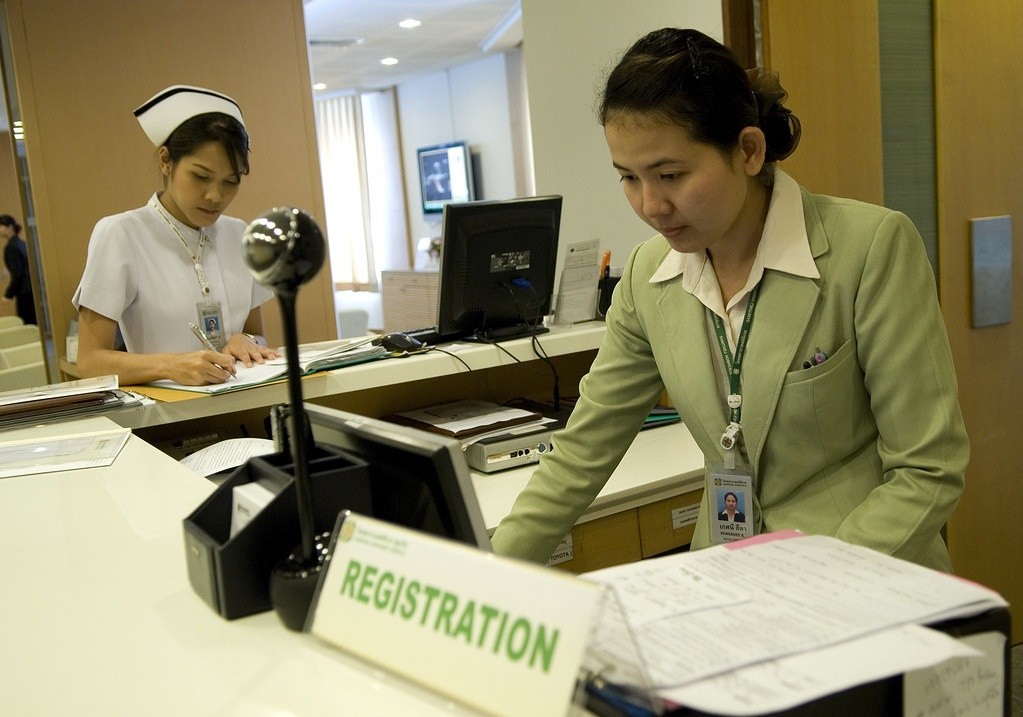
[380,334,421,352]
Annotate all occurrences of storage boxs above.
[181,443,369,620]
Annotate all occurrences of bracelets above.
[242,333,258,345]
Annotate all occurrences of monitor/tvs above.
[417,142,478,214]
[298,403,493,550]
[435,194,562,345]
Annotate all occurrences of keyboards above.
[371,327,438,347]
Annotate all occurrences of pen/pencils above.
[803,345,828,369]
[600,250,611,278]
[189,323,238,380]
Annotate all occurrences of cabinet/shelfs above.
[382,271,439,333]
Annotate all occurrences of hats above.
[133,84,248,147]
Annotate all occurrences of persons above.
[71,84,283,387]
[486,26,971,578]
[717,493,745,523]
[207,318,219,336]
[0,214,38,327]
